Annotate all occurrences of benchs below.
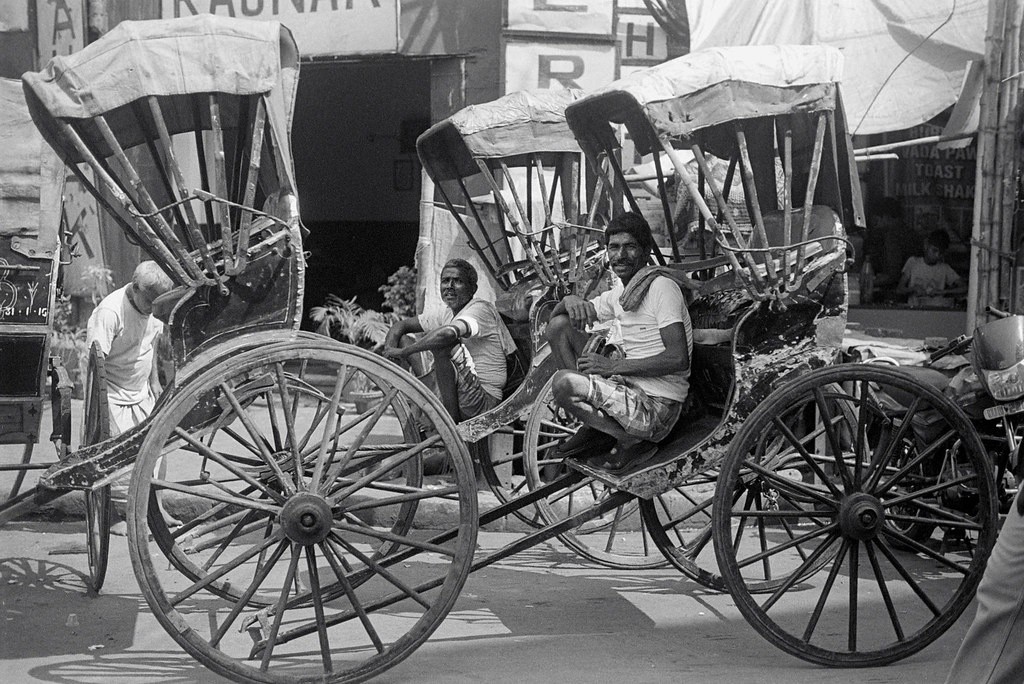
[147,186,306,324]
[683,202,848,347]
[494,212,609,322]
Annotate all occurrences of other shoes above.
[587,440,658,474]
[562,427,616,458]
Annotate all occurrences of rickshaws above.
[180,91,764,568]
[241,43,998,669]
[0,78,111,592]
[0,12,306,683]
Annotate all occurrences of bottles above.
[862,261,874,303]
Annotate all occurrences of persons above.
[546,212,693,474]
[896,229,967,307]
[944,480,1024,684]
[78,260,183,536]
[382,258,508,432]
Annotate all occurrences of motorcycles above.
[856,314,1024,560]
